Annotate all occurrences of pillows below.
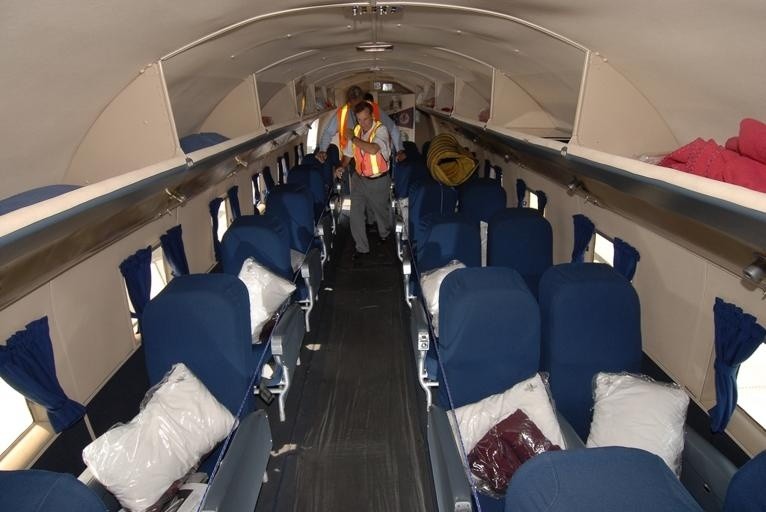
[587,372,688,482]
[80,363,239,512]
[444,374,567,497]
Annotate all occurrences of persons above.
[316,85,408,265]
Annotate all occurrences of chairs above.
[390,141,553,498]
[539,262,672,442]
[1,470,109,512]
[217,141,344,424]
[505,446,705,512]
[142,273,256,475]
[722,448,765,512]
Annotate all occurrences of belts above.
[364,172,387,180]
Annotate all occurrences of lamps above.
[353,0,401,53]
